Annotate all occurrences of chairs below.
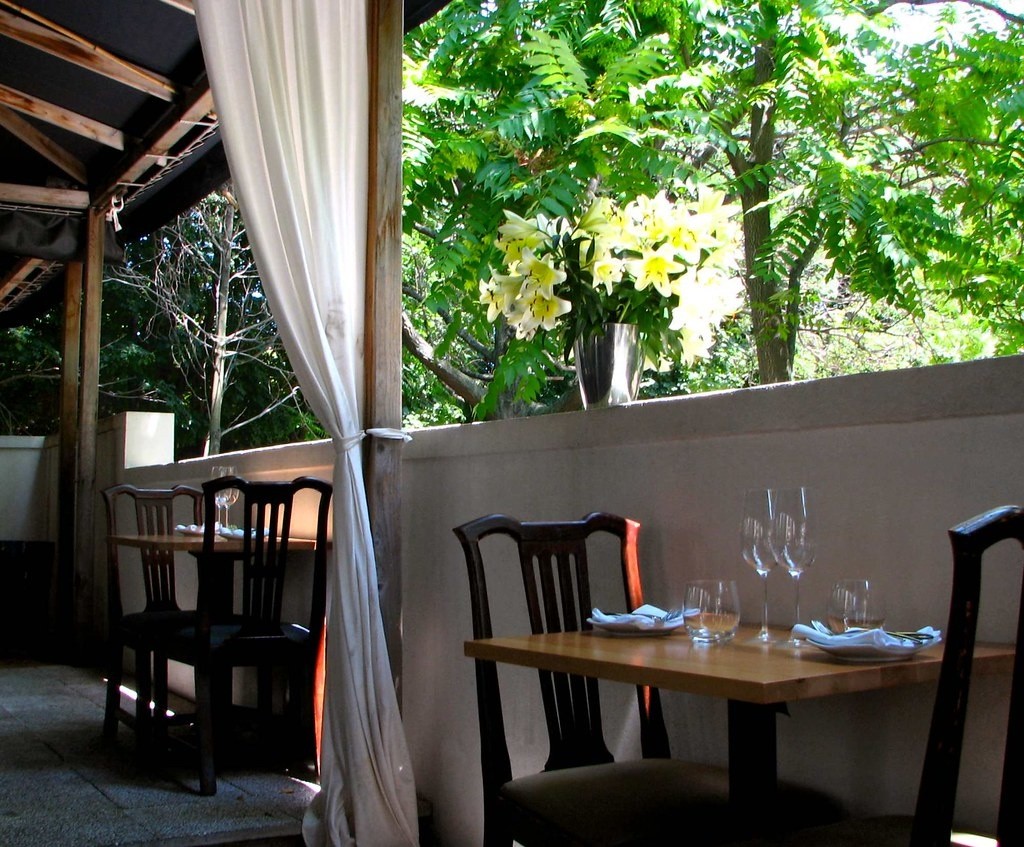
[452,513,783,847]
[100,483,233,761]
[703,501,1024,847]
[153,475,332,797]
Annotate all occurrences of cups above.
[683,580,740,644]
[828,579,886,634]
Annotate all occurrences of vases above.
[571,322,643,410]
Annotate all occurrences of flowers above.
[476,190,743,343]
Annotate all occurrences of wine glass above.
[210,466,239,532]
[769,486,817,648]
[742,488,782,644]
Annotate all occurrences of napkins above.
[176,521,221,533]
[791,624,942,652]
[220,527,269,535]
[589,603,701,632]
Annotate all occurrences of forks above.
[811,620,923,645]
[603,608,682,621]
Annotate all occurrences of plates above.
[587,618,683,636]
[218,534,267,541]
[805,637,941,661]
[174,528,204,537]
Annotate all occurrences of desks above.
[108,531,331,785]
[463,621,1019,847]
